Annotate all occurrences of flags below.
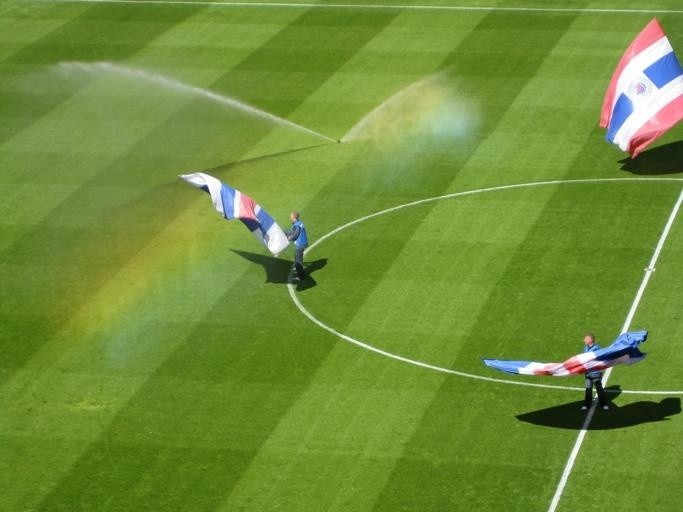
[176,170,290,260]
[480,328,648,380]
[597,18,683,160]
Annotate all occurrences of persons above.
[283,210,308,281]
[578,333,610,410]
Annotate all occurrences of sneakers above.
[291,266,305,281]
[581,405,609,412]
